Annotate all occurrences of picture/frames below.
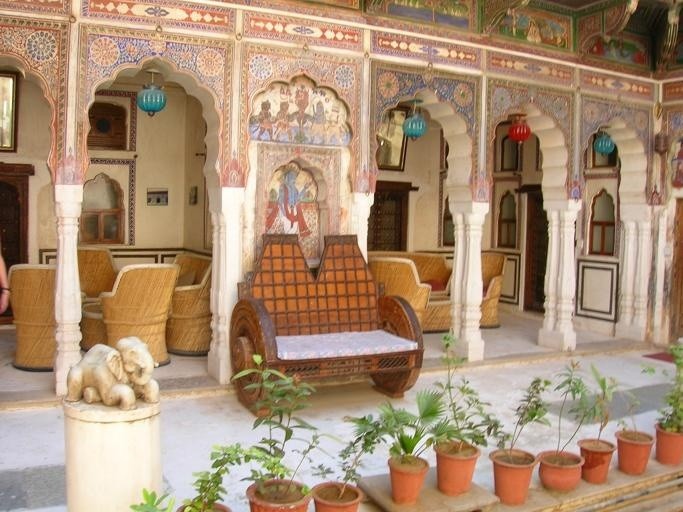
[377,107,410,171]
[0,70,19,152]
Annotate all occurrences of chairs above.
[367,251,507,333]
[8,246,212,372]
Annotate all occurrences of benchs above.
[230,235,424,417]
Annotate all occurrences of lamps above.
[403,100,427,140]
[137,69,166,116]
[509,114,530,147]
[594,125,614,157]
